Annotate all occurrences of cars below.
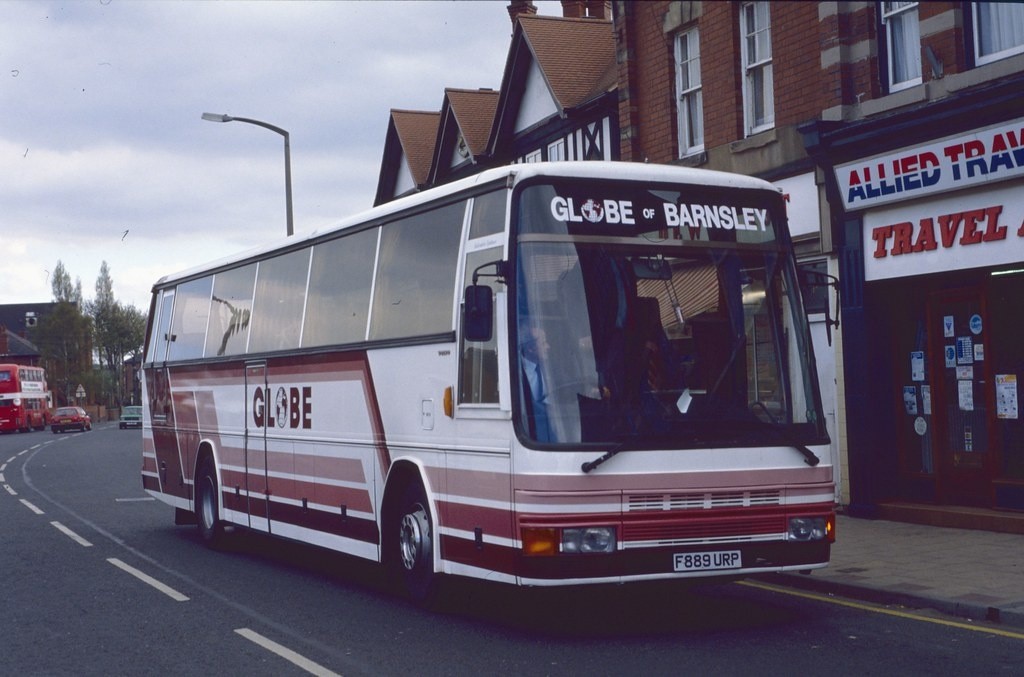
[118,405,143,429]
[49,406,95,435]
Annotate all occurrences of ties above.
[535,364,544,401]
[616,259,638,336]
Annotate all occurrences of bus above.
[139,159,842,607]
[0,364,50,435]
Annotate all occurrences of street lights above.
[200,110,297,237]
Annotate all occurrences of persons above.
[519,327,610,404]
[629,340,684,390]
